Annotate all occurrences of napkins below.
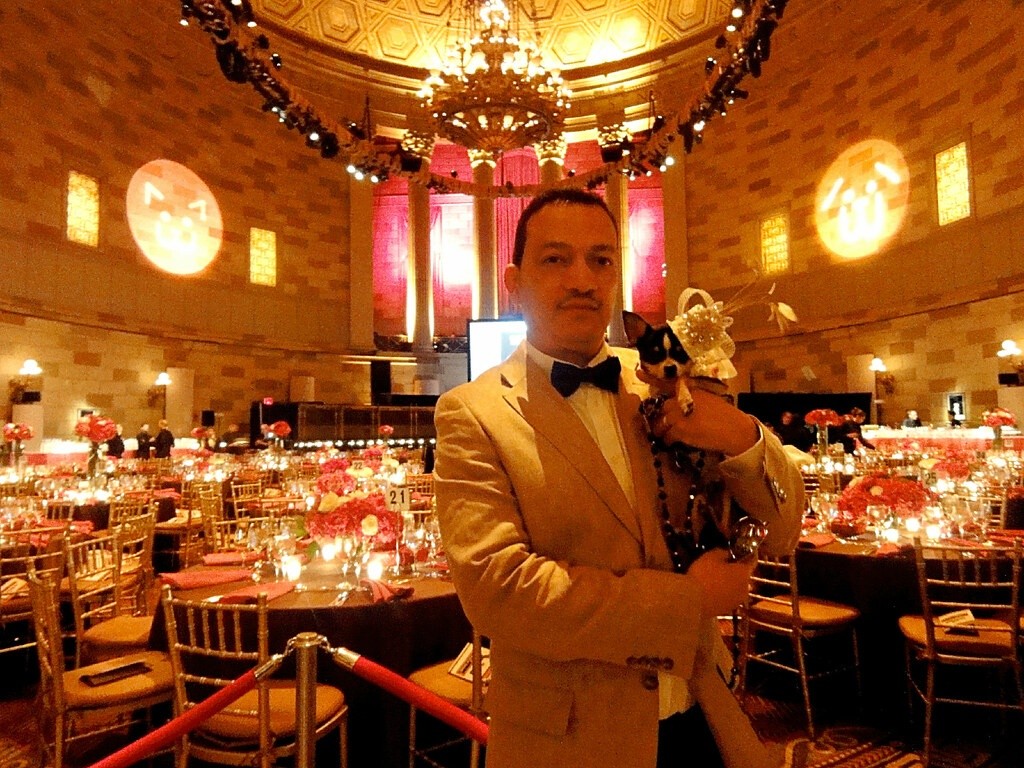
[798,533,832,548]
[946,538,991,559]
[199,553,263,566]
[367,578,415,603]
[219,581,298,604]
[160,568,257,589]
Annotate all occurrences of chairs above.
[1,445,1024,767]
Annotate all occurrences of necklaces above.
[636,393,709,570]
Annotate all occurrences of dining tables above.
[774,504,1024,673]
[147,550,477,768]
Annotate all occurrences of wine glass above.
[0,449,443,590]
[786,415,1024,546]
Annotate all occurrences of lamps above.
[870,357,898,396]
[8,359,44,404]
[999,339,1024,385]
[146,372,171,406]
[172,0,782,199]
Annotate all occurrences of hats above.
[158,420,171,430]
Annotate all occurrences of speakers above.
[400,157,422,171]
[602,146,623,162]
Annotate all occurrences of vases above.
[816,429,830,463]
[273,436,288,465]
[89,440,105,480]
[990,427,1006,462]
[8,437,25,471]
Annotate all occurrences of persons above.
[106,423,124,458]
[214,423,269,454]
[900,409,922,429]
[828,408,876,454]
[948,411,961,425]
[764,411,794,444]
[149,419,174,459]
[136,422,154,458]
[432,188,806,768]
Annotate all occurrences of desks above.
[868,436,1024,454]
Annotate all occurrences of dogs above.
[621,310,744,547]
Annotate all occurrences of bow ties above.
[549,355,623,398]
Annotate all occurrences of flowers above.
[1,415,411,567]
[839,470,927,516]
[982,408,1019,434]
[805,410,845,431]
[669,275,796,371]
